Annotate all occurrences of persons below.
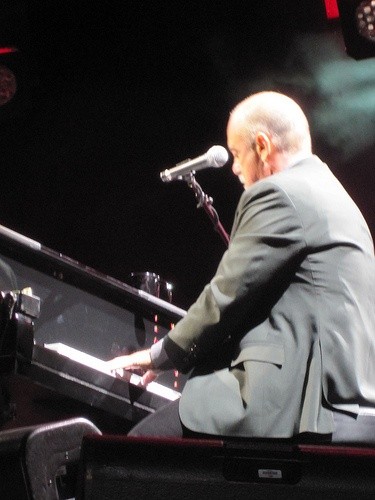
[104,92,374,443]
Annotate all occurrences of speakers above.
[0,418,103,500]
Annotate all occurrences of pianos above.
[1,222,244,499]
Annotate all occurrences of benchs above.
[78,434,375,500]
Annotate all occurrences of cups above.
[159,281,173,305]
[128,272,160,298]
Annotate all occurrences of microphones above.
[160,145,228,182]
